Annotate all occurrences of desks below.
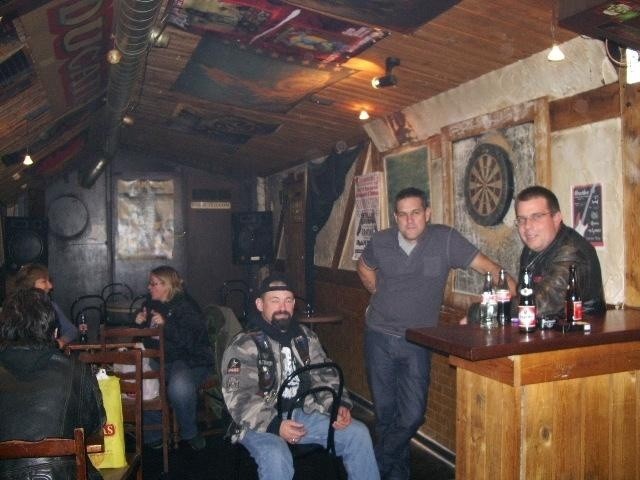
[403,307,639,480]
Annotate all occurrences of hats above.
[255,271,299,298]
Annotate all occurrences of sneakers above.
[150,431,174,449]
[187,434,207,452]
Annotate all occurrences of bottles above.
[78,315,88,344]
[565,264,583,330]
[517,267,538,333]
[532,275,547,320]
[479,271,498,329]
[495,268,512,326]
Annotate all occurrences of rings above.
[289,438,299,445]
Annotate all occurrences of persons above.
[460,187,609,327]
[0,286,109,479]
[126,264,214,454]
[355,185,519,479]
[3,260,81,350]
[221,274,383,480]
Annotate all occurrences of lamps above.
[370,54,403,90]
[547,1,567,64]
[22,120,35,166]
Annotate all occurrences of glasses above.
[513,210,564,226]
